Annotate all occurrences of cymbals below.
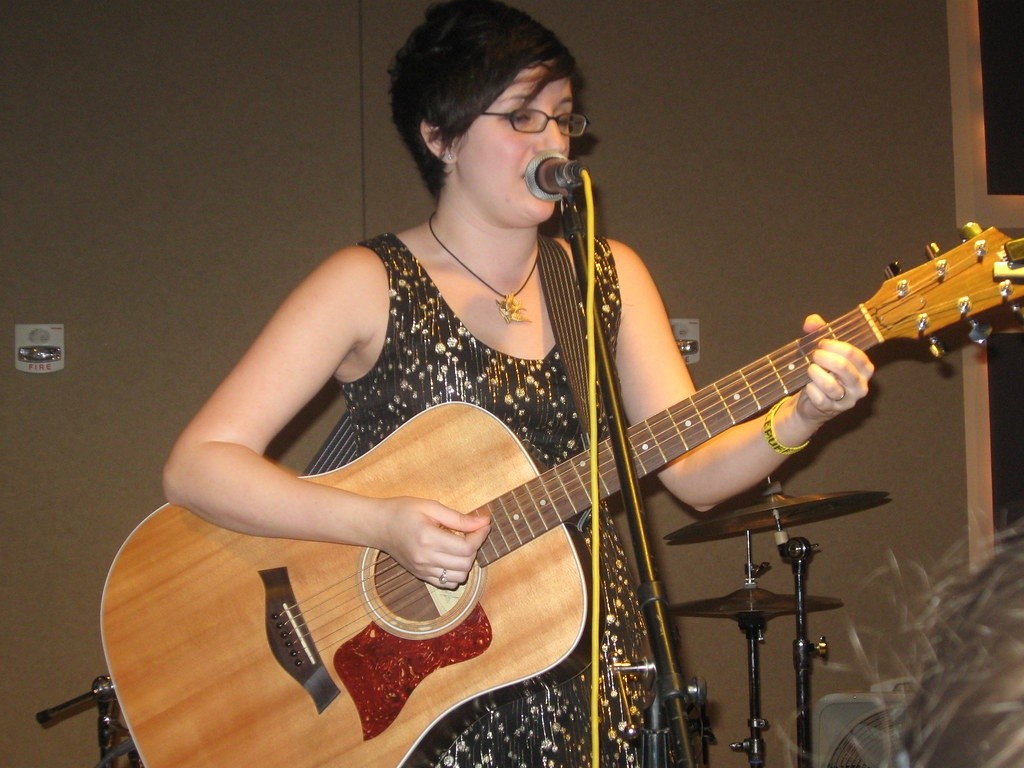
[665,488,893,548]
[669,587,845,620]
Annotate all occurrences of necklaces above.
[430,212,537,323]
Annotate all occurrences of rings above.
[837,386,847,401]
[438,568,448,583]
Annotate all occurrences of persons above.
[159,0,877,768]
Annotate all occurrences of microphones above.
[525,149,590,201]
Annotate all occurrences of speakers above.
[813,691,917,768]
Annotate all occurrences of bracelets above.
[764,396,811,455]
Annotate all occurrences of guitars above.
[100,223,1021,767]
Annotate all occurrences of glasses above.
[481,108,591,138]
